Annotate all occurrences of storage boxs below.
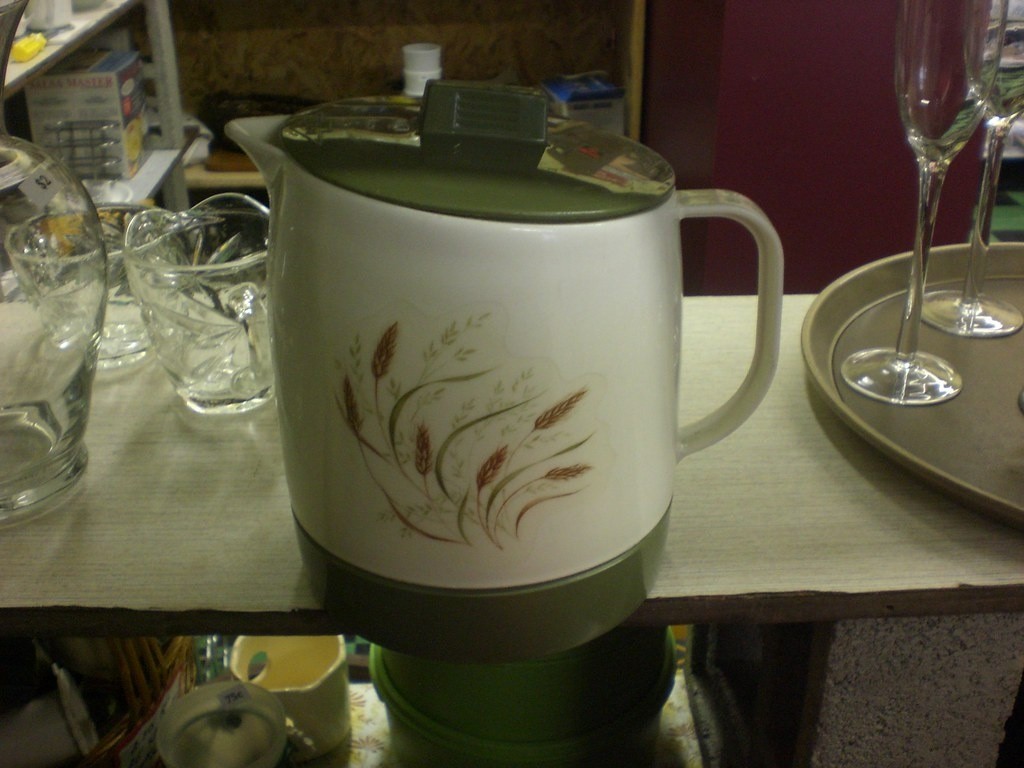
[540,77,628,138]
[23,51,153,179]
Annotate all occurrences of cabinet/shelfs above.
[0,0,197,254]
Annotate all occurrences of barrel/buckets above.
[366,626,675,768]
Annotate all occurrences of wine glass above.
[919,0,1024,338]
[836,0,1008,405]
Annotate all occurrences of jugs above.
[226,82,782,666]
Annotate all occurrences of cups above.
[124,193,276,417]
[5,201,168,373]
[154,681,315,768]
[229,634,351,761]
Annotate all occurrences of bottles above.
[0,1,108,534]
[400,42,442,97]
